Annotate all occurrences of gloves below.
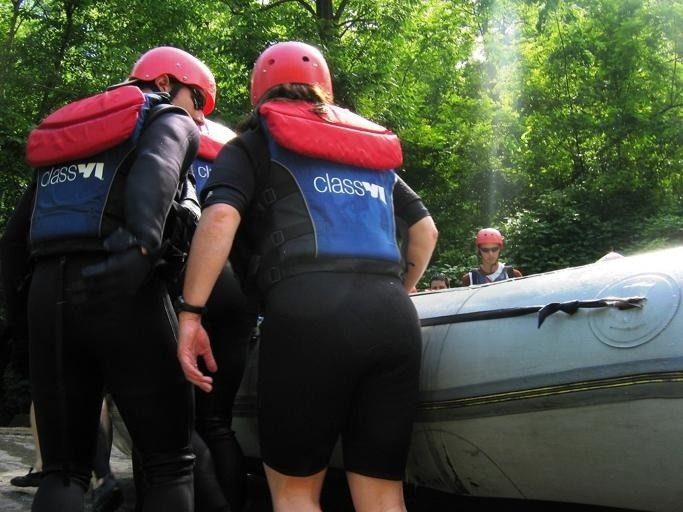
[89,245,152,304]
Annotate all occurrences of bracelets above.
[173,295,207,318]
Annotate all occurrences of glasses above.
[170,77,205,110]
[479,246,499,252]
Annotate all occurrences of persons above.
[171,38,440,511]
[0,41,217,510]
[125,111,277,512]
[460,227,523,287]
[428,275,449,291]
[8,393,129,510]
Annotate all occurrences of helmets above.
[251,41,334,108]
[128,47,217,116]
[477,228,503,249]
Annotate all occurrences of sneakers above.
[10,468,43,487]
[89,476,121,511]
